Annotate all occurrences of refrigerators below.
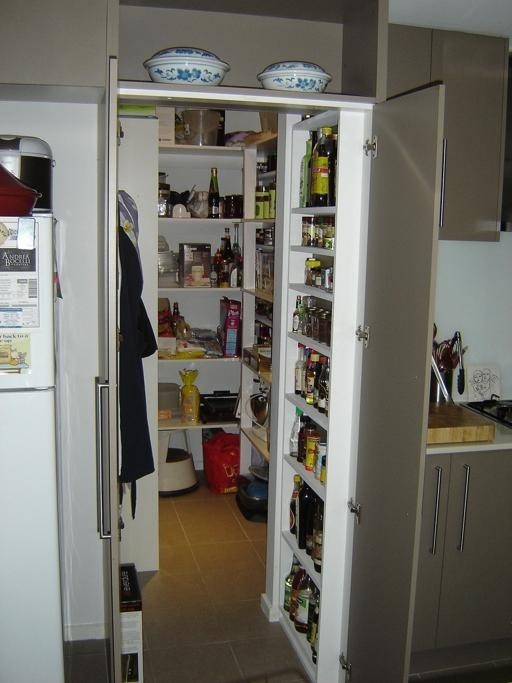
[0,214,66,683]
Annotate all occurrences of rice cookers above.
[0,132,55,214]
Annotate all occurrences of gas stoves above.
[467,394,512,426]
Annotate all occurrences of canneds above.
[320,267,330,290]
[224,195,240,218]
[315,443,328,480]
[305,306,331,347]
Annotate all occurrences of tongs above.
[446,330,464,394]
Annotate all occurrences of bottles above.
[172,300,191,337]
[208,165,243,289]
[255,180,275,345]
[283,125,338,665]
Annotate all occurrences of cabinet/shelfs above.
[1,0,107,102]
[387,21,509,242]
[409,404,512,681]
[277,78,448,683]
[157,144,245,432]
[240,137,278,464]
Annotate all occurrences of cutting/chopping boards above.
[427,399,495,444]
